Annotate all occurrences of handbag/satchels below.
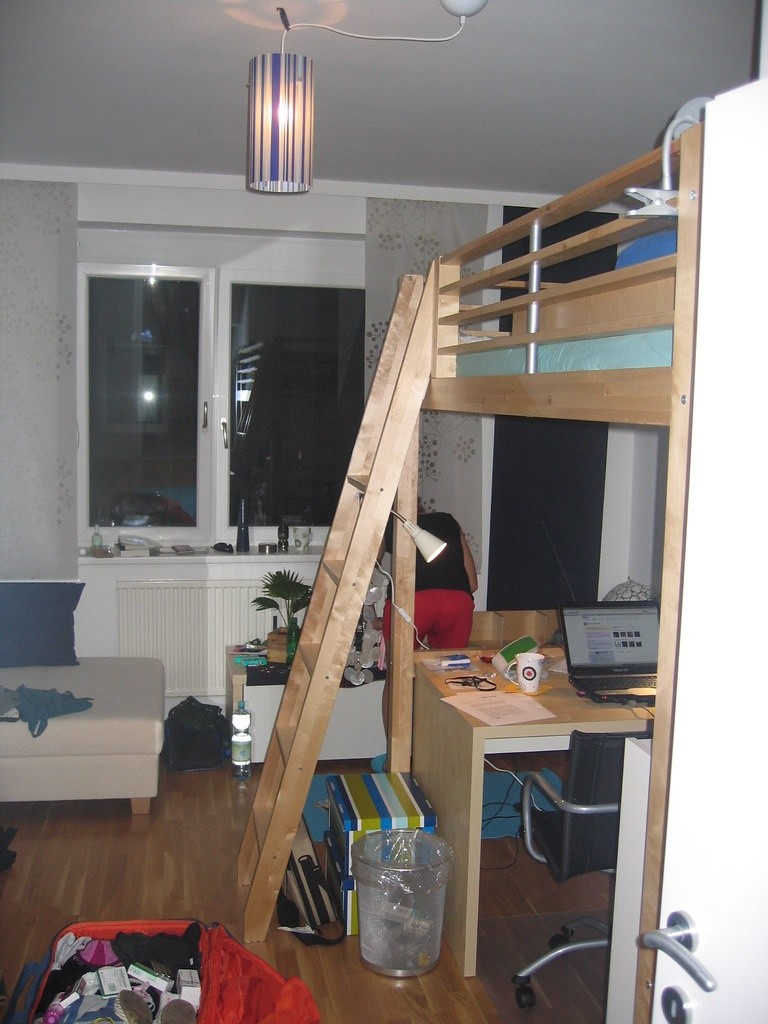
[276,813,347,947]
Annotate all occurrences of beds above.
[419,126,703,426]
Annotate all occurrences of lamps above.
[361,493,447,562]
[247,54,314,193]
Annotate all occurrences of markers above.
[273,616,277,630]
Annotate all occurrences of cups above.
[293,527,312,552]
[506,652,544,693]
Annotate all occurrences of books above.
[114,539,149,557]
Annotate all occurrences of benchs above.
[0,656,165,815]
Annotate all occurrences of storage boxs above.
[267,627,302,663]
[59,977,86,1008]
[97,961,201,1006]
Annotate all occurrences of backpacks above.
[163,695,233,772]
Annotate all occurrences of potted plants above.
[251,568,312,663]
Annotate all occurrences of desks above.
[223,646,246,736]
[413,647,655,976]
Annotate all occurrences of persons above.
[372,497,478,773]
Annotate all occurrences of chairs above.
[512,730,654,1008]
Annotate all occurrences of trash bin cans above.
[352,828,453,978]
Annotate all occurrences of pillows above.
[0,581,85,667]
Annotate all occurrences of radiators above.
[116,578,312,697]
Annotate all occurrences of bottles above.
[286,617,300,665]
[231,701,251,782]
[236,499,249,552]
[92,523,102,548]
[43,992,65,1024]
[278,519,289,552]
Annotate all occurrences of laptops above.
[556,600,659,703]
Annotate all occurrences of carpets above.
[302,767,563,842]
